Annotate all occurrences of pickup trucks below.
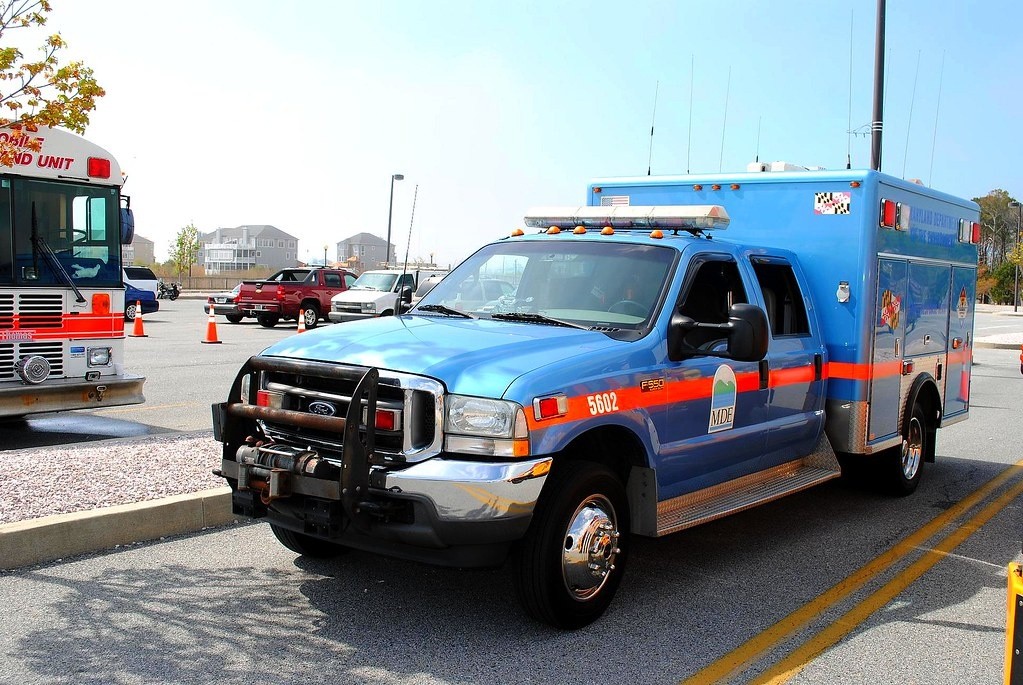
[210,168,982,632]
[238,265,359,329]
[327,270,419,323]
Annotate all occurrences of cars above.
[203,280,243,324]
[124,284,160,322]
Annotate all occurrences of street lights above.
[385,174,404,270]
[323,245,329,267]
[429,252,435,263]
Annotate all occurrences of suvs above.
[123,265,159,299]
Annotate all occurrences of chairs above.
[687,269,737,325]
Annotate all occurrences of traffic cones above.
[298,309,307,334]
[129,301,149,338]
[201,304,222,344]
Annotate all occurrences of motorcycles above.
[156,281,180,301]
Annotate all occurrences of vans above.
[409,277,517,312]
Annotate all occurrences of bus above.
[0,119,147,417]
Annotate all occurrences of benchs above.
[760,285,801,336]
